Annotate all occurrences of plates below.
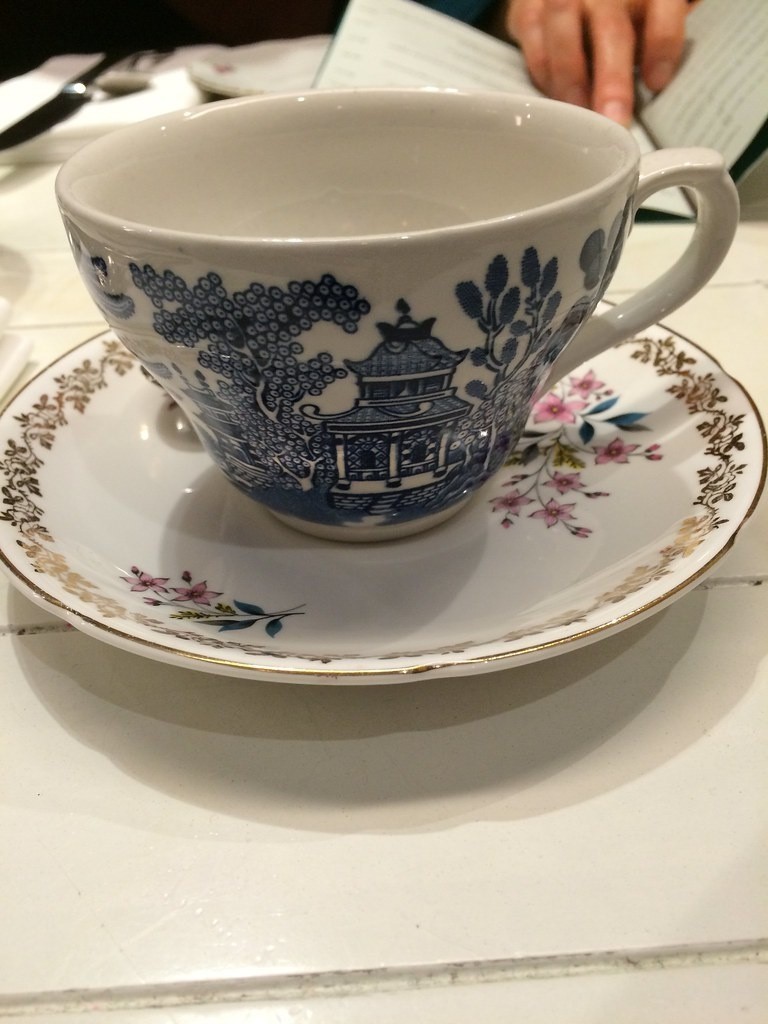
[0,328,768,688]
[191,36,335,97]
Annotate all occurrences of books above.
[312,0,768,219]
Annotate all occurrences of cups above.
[52,87,741,544]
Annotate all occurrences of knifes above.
[0,54,123,151]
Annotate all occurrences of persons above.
[420,0,688,127]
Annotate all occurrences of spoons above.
[96,46,175,95]
